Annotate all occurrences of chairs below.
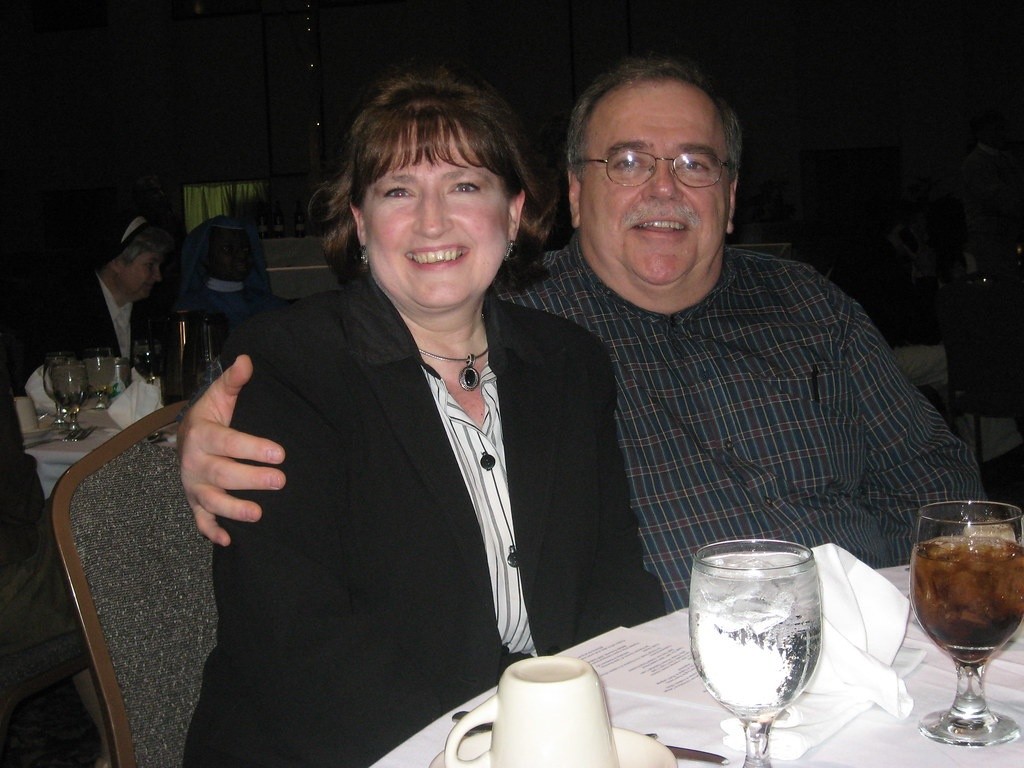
[49,399,221,768]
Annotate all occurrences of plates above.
[429,727,676,768]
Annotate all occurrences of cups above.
[444,656,618,768]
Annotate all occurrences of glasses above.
[572,149,731,188]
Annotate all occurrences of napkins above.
[797,542,915,722]
[107,379,165,429]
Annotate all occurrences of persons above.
[21,216,288,381]
[179,133,667,768]
[180,56,987,768]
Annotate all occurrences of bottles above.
[294,201,305,238]
[272,201,284,239]
[257,198,271,239]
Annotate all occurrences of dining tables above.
[19,393,128,500]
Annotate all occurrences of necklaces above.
[419,343,487,391]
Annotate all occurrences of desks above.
[366,564,1024,768]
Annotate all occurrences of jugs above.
[145,309,231,407]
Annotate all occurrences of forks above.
[24,426,98,448]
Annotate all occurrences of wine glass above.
[909,501,1024,749]
[44,339,164,435]
[688,539,821,768]
